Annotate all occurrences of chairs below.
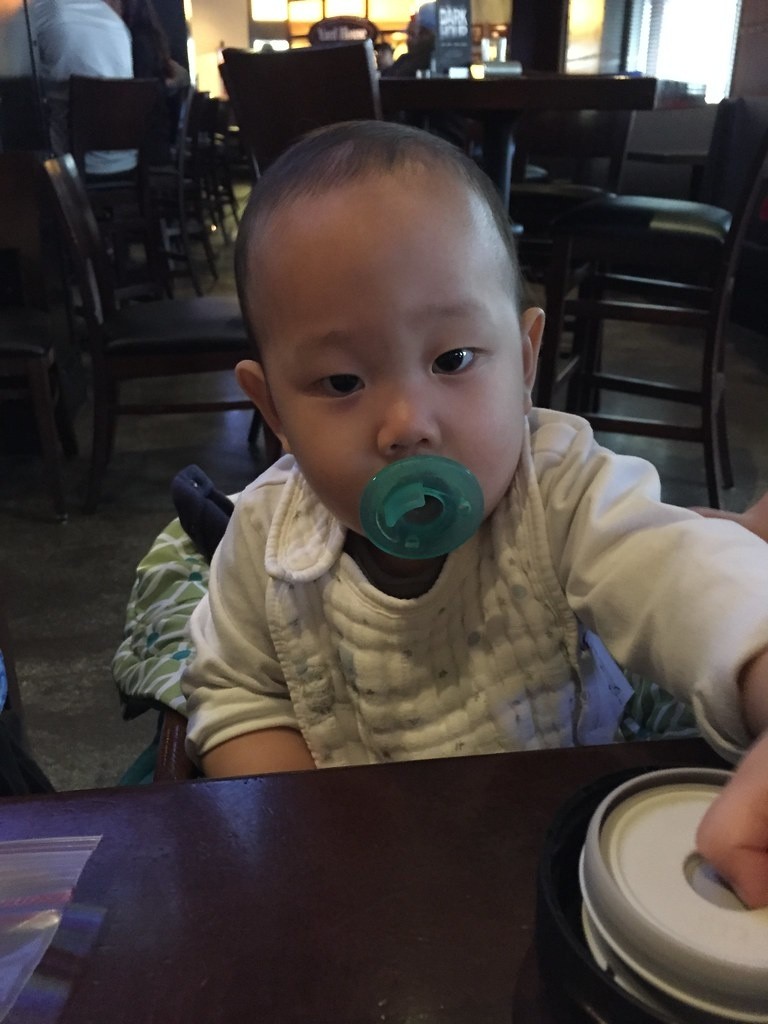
[1,15,768,509]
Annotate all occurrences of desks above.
[0,734,768,1024]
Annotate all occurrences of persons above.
[373,4,437,78]
[0,1,190,180]
[180,120,767,912]
